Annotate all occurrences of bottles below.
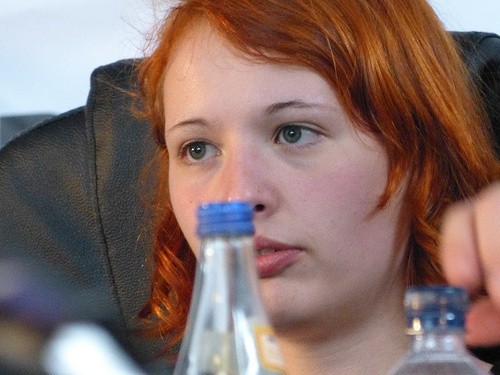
[173,199,290,375]
[387,285,485,375]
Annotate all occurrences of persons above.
[128,0,500,375]
